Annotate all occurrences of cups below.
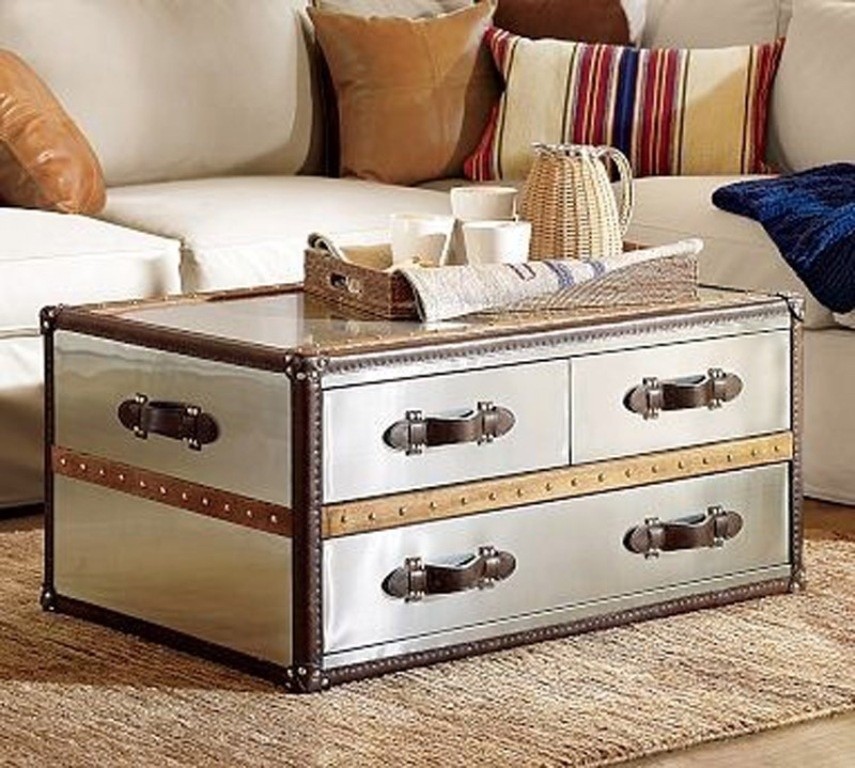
[388,213,454,268]
[450,186,518,223]
[460,220,533,267]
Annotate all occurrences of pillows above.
[307,1,786,184]
[0,50,107,217]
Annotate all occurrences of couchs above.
[1,1,855,508]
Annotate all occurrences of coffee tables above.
[38,280,805,693]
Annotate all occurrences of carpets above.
[0,529,854,767]
